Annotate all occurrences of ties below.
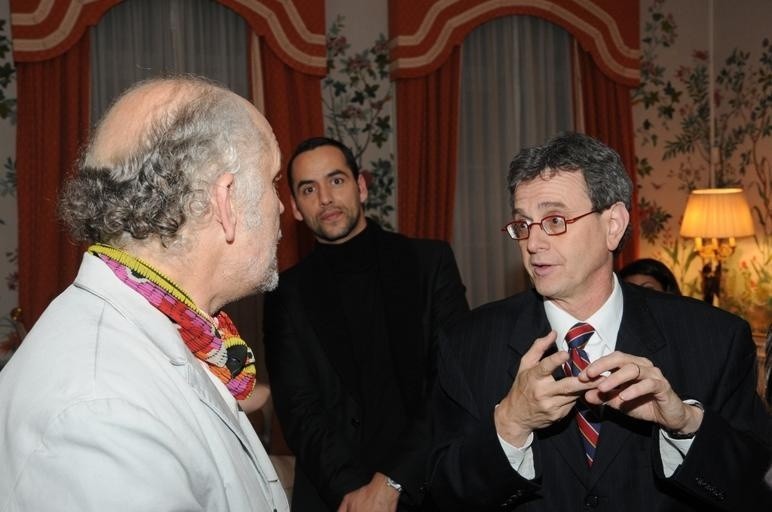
[563,323,602,470]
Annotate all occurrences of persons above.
[411,127,772,512]
[0,75,299,512]
[616,256,683,301]
[262,134,474,512]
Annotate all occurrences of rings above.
[632,362,643,380]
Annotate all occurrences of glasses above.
[501,202,615,240]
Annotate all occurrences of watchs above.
[384,476,403,492]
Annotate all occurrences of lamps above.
[680,187,757,304]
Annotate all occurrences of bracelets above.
[667,402,706,441]
